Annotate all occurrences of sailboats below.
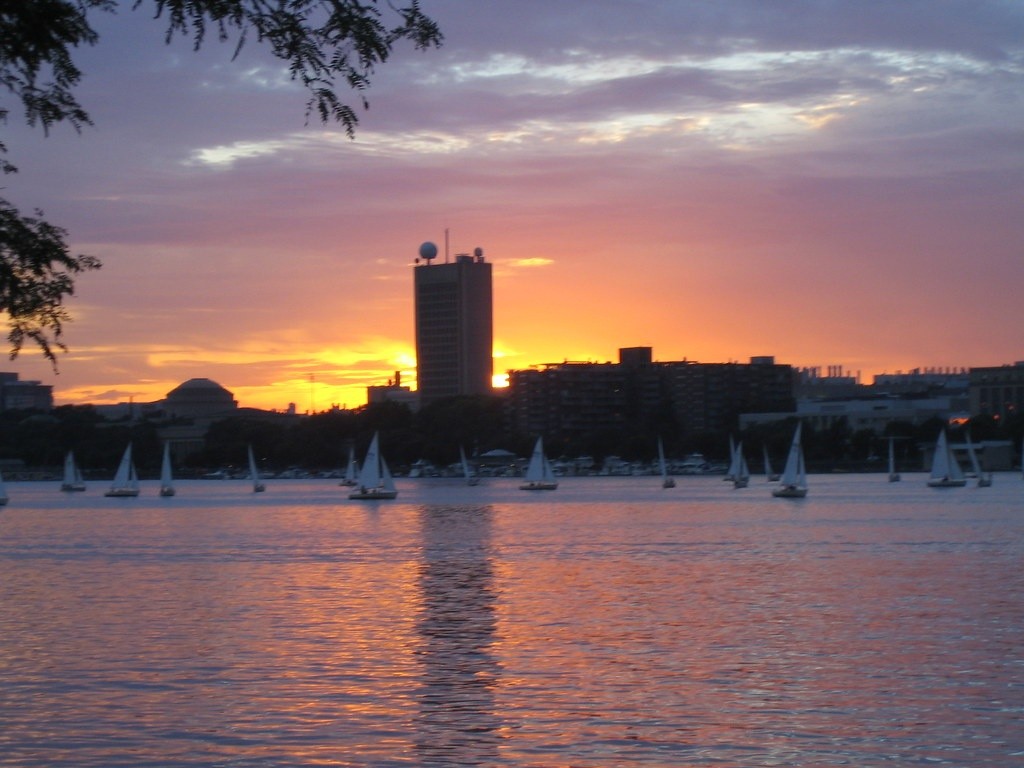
[723,434,736,482]
[656,436,676,487]
[104,441,140,496]
[459,444,482,486]
[59,450,87,492]
[246,448,266,492]
[926,426,968,487]
[734,443,749,488]
[160,440,175,496]
[348,430,397,500]
[771,420,809,498]
[888,436,900,482]
[761,445,781,481]
[518,437,558,490]
[339,449,361,485]
[963,429,982,477]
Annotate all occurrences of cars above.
[269,465,347,479]
[407,460,463,478]
[666,460,726,475]
[549,456,659,477]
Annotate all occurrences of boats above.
[976,477,993,486]
[0,482,9,505]
